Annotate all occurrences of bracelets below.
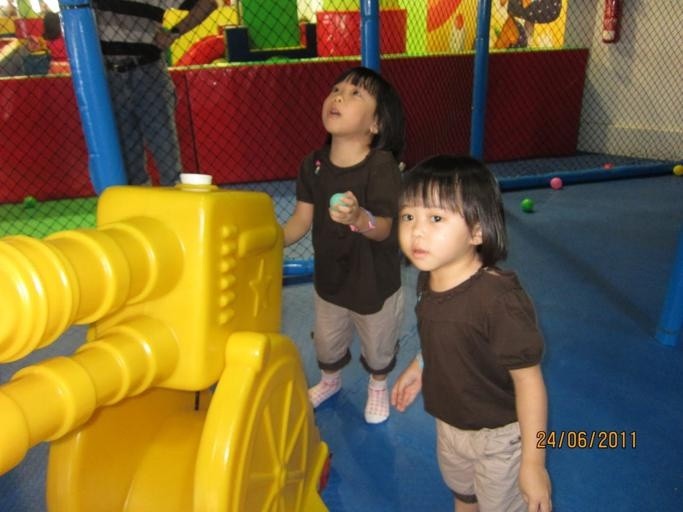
[416,354,424,368]
[171,26,180,40]
[348,208,377,233]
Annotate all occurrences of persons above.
[392,153,554,511]
[89,1,221,191]
[277,66,407,425]
[41,12,68,63]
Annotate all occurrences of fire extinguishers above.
[602,0,623,42]
